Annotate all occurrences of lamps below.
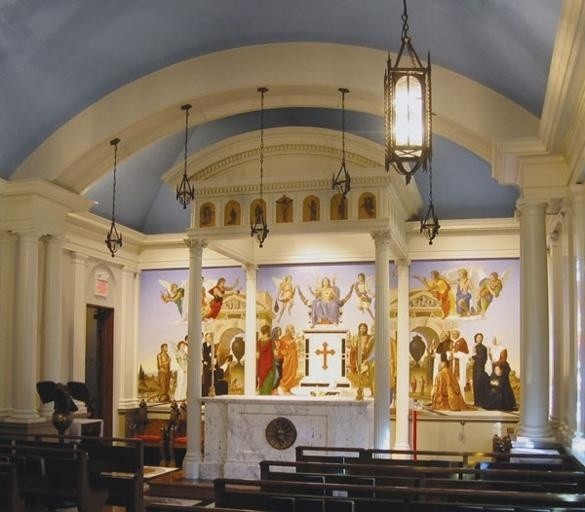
[382,0,432,185]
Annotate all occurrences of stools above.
[127,400,204,468]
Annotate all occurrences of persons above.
[307,275,339,324]
[201,276,244,322]
[426,268,452,317]
[199,275,207,315]
[354,272,375,320]
[449,268,475,317]
[275,273,298,322]
[157,281,186,318]
[476,271,504,317]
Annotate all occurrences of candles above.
[211,335,215,366]
[357,335,362,369]
